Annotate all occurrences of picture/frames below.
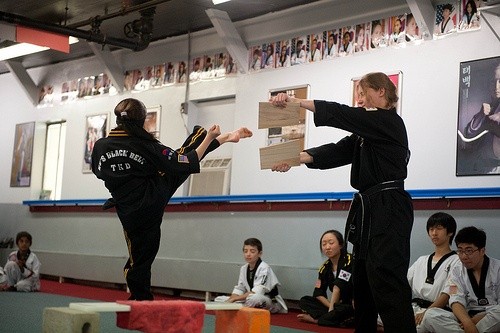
[82,111,110,175]
[143,105,162,140]
[456,55,500,177]
[350,71,403,117]
[265,83,310,150]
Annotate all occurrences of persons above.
[421,226,500,333]
[269,73,418,333]
[296,230,354,329]
[407,212,459,331]
[91,98,253,301]
[214,238,288,314]
[463,63,500,174]
[0,232,41,292]
[37,0,482,106]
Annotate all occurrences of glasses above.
[455,248,479,255]
